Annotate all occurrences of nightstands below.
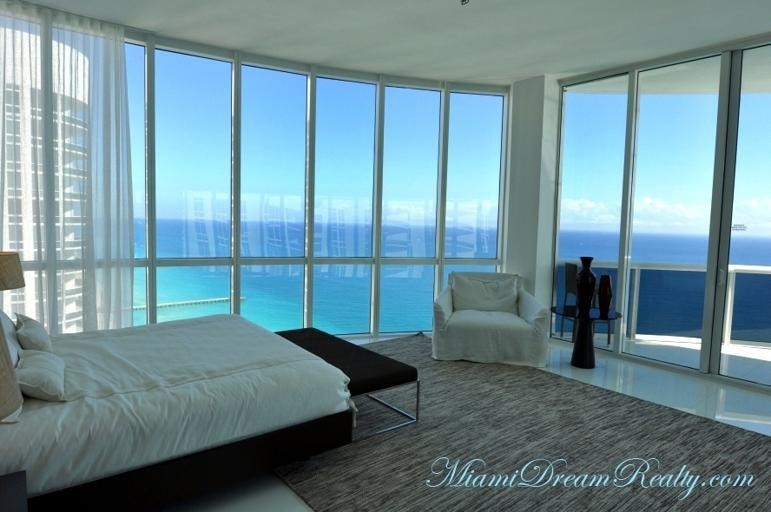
[0,470,29,511]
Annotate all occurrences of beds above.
[0,307,359,500]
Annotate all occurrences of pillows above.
[451,271,521,316]
[0,313,67,424]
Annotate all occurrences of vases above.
[576,257,596,316]
[598,275,613,315]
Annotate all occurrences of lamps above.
[0,319,25,419]
[0,251,25,313]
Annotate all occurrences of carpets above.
[252,332,770,511]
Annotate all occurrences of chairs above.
[548,305,622,370]
[561,262,611,344]
[431,271,551,369]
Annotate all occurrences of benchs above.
[271,327,421,441]
